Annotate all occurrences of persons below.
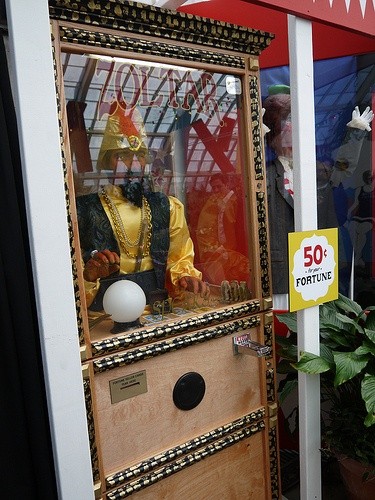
[80,96,211,317]
[257,86,375,369]
